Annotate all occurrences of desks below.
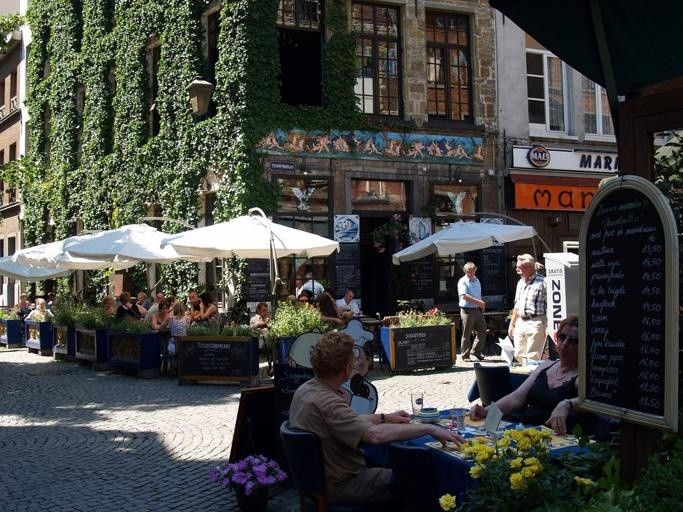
[396,409,600,506]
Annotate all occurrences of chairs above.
[388,442,439,511]
[278,418,368,512]
[474,364,513,406]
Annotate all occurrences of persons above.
[250,273,362,334]
[16,292,56,320]
[508,254,547,362]
[102,289,217,354]
[457,262,486,362]
[469,315,578,436]
[287,331,468,512]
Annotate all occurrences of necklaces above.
[554,363,577,381]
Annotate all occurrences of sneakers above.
[476,353,484,360]
[464,358,471,362]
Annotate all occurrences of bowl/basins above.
[417,407,440,423]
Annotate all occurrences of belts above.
[521,314,538,320]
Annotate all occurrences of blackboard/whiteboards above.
[578,174,680,434]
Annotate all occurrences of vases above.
[233,489,273,512]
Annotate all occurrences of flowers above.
[210,454,289,495]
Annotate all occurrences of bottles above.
[449,408,466,433]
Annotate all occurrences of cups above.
[409,392,422,414]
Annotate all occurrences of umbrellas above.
[156,205,343,296]
[1,255,78,284]
[62,214,222,312]
[391,209,554,270]
[10,234,153,297]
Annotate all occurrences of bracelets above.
[377,412,387,426]
[563,398,575,411]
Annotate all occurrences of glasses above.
[300,300,309,302]
[559,333,578,345]
